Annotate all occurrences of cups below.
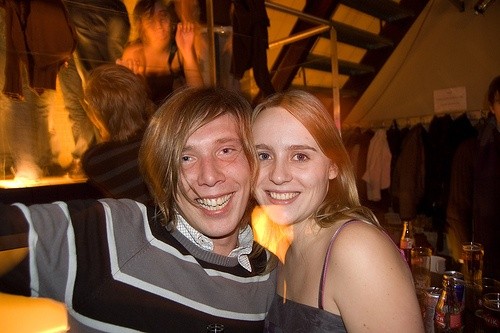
[482,286,500,333]
[411,247,432,289]
[462,242,483,288]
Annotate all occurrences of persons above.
[0,0,75,181]
[0,84,281,333]
[447,76,500,281]
[115,0,206,110]
[252,90,425,333]
[79,65,157,209]
[57,0,131,179]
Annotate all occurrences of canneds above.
[439,270,465,323]
[424,286,443,333]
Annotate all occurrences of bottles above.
[434,275,462,333]
[400,221,415,268]
